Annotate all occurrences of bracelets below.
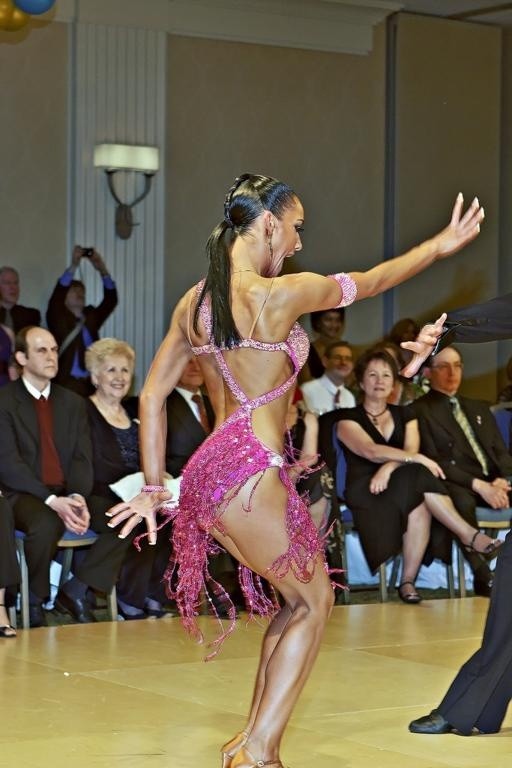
[141,485,165,493]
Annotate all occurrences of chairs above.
[331,425,456,603]
[456,507,511,598]
[490,400,511,448]
[14,524,123,627]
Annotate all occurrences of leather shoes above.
[116,600,174,619]
[409,709,456,733]
[54,588,97,622]
[28,601,48,628]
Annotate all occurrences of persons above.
[105,173,485,768]
[282,308,512,606]
[1,324,281,637]
[1,246,120,399]
[400,292,512,736]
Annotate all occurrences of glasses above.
[430,361,464,370]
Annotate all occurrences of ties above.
[452,396,490,477]
[192,394,209,431]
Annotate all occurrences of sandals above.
[0,604,17,637]
[464,530,504,560]
[398,581,422,603]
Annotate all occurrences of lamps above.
[93,141,158,239]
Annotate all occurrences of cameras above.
[81,247,95,259]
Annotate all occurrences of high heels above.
[220,733,283,768]
